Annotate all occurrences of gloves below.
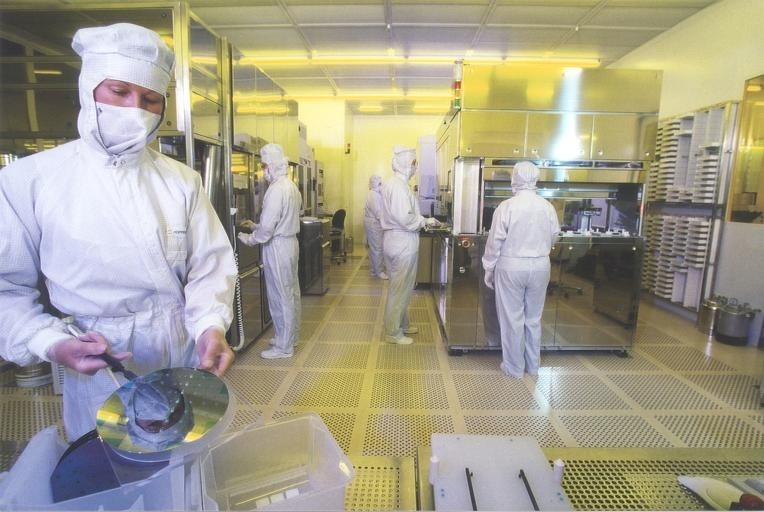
[239,219,254,228]
[425,217,442,227]
[237,231,248,246]
[483,270,494,289]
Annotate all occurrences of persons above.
[0,21,239,444]
[237,142,303,359]
[363,174,389,281]
[379,146,442,346]
[481,161,562,381]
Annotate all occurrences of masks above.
[409,165,416,177]
[378,185,382,191]
[95,101,162,159]
[262,168,273,183]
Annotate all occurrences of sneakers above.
[403,325,420,334]
[383,330,414,346]
[376,268,388,280]
[268,336,299,348]
[499,361,525,381]
[259,346,295,358]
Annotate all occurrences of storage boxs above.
[201,412,354,512]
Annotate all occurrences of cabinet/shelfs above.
[298,219,331,295]
[476,242,637,347]
